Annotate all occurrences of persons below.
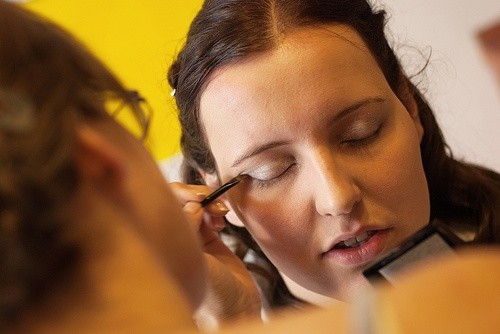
[166,0,499,322]
[0,0,500,334]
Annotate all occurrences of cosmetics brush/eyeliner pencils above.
[200,172,251,210]
